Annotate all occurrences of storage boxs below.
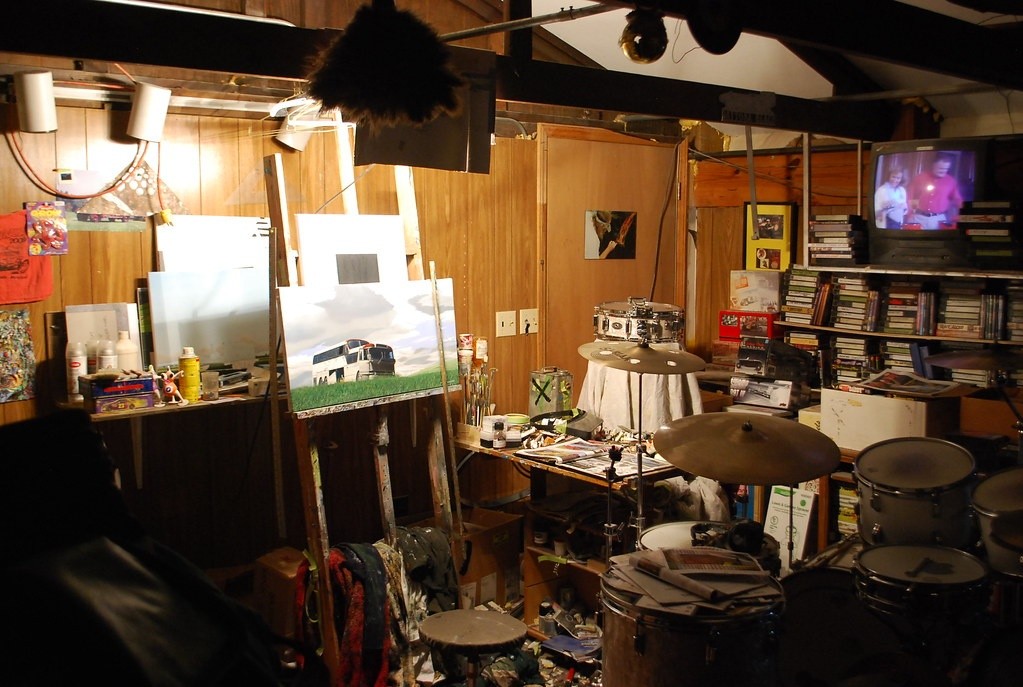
[699,387,733,414]
[722,404,793,420]
[406,506,525,614]
[765,486,820,581]
[798,385,1023,452]
[729,376,802,409]
[253,545,309,665]
[718,269,782,377]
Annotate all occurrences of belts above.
[916,210,945,217]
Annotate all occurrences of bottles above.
[65,341,88,394]
[179,346,201,404]
[493,422,506,449]
[87,331,138,375]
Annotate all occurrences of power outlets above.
[519,309,539,334]
[496,311,517,338]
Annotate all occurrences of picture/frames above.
[741,200,797,278]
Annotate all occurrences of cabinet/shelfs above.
[691,143,873,363]
[454,431,685,667]
[773,262,1023,399]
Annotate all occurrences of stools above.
[418,608,528,687]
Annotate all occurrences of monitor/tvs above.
[868,137,1000,269]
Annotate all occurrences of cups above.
[554,537,568,556]
[534,530,548,544]
[201,371,220,401]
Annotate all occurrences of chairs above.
[0,408,332,687]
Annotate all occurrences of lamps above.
[13,69,60,133]
[275,93,324,153]
[126,81,172,143]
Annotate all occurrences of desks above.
[818,376,1023,556]
[575,337,703,434]
[88,386,288,499]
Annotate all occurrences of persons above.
[874,165,905,229]
[907,154,964,231]
[593,211,630,259]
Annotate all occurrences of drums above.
[635,519,782,581]
[776,534,942,687]
[598,551,792,687]
[855,543,990,619]
[851,436,976,547]
[593,299,686,344]
[971,467,1023,579]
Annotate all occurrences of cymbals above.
[577,341,706,375]
[654,412,842,485]
